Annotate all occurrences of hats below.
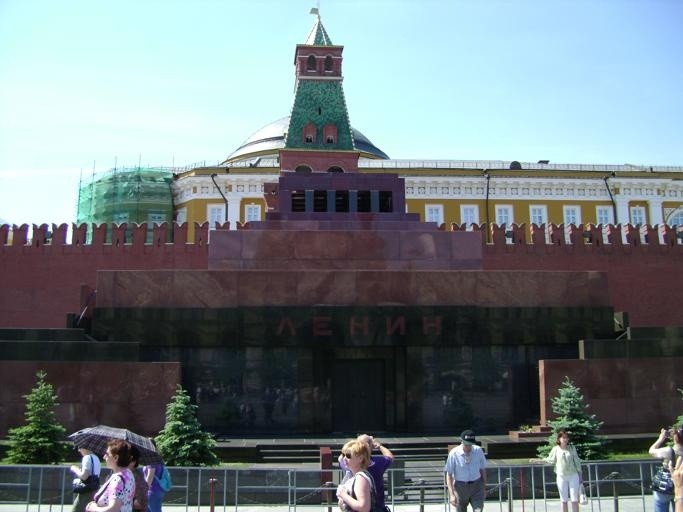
[459,430,478,445]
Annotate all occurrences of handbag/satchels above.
[649,466,674,494]
[579,483,587,505]
[72,474,99,493]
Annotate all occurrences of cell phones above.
[664,426,670,437]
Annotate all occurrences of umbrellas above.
[67,425,162,465]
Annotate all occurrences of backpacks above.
[159,469,172,492]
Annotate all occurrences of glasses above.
[342,453,351,458]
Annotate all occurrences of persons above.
[531,430,583,512]
[338,434,395,512]
[336,440,377,512]
[70,439,165,511]
[443,430,487,512]
[649,427,683,512]
